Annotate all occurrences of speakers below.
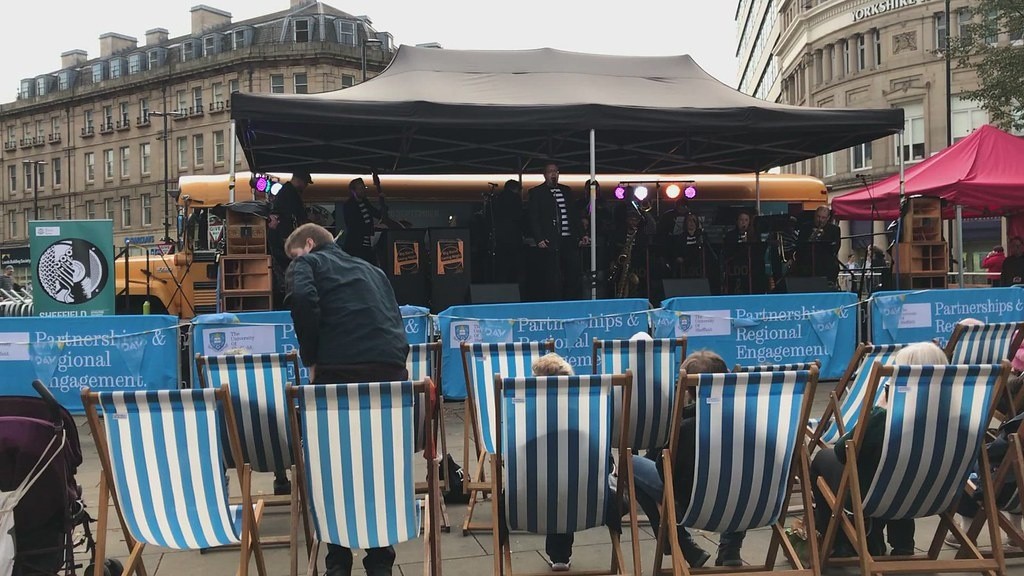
[767,275,838,295]
[656,278,711,300]
[467,283,521,305]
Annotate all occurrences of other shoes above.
[550,556,572,570]
[1007,537,1020,548]
[945,533,979,549]
[716,551,742,565]
[685,546,712,567]
[832,549,854,560]
[272,479,290,495]
[889,545,915,557]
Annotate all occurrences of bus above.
[109,166,840,319]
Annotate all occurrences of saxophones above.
[613,226,638,297]
[792,222,825,260]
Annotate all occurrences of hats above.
[294,168,314,185]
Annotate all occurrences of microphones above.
[489,182,498,187]
[856,174,871,178]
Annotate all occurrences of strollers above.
[0,379,126,576]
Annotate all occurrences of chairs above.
[82,322,1024,576]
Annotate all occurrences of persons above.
[267,168,314,311]
[0,265,15,302]
[866,244,889,299]
[945,329,1024,550]
[532,352,616,570]
[627,351,747,568]
[981,245,1007,287]
[493,165,642,301]
[679,207,841,294]
[284,223,411,576]
[842,252,860,274]
[1000,236,1024,287]
[342,178,389,268]
[809,341,949,556]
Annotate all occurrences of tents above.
[228,45,905,301]
[831,125,1023,288]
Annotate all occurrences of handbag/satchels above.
[437,455,473,504]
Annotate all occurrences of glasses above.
[354,186,369,190]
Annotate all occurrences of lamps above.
[181,195,192,207]
[250,173,282,196]
[166,189,181,203]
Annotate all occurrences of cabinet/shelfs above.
[899,274,948,290]
[904,197,941,242]
[180,203,273,319]
[892,242,947,273]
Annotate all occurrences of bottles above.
[143,301,150,315]
